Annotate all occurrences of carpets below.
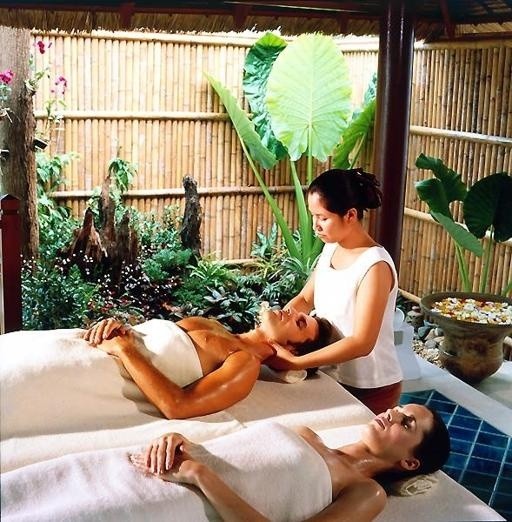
[399,389,512,522]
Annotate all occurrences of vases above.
[421,291,512,386]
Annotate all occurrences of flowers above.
[40,75,67,143]
[30,40,53,87]
[0,69,15,109]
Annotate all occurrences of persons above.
[1,304,332,434]
[260,166,405,418]
[1,401,451,522]
